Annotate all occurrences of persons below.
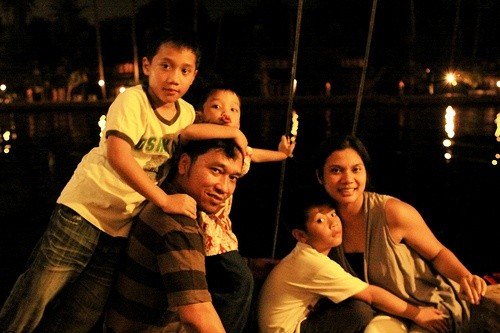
[101,87,296,333]
[0,27,200,333]
[316,133,487,333]
[256,204,451,333]
